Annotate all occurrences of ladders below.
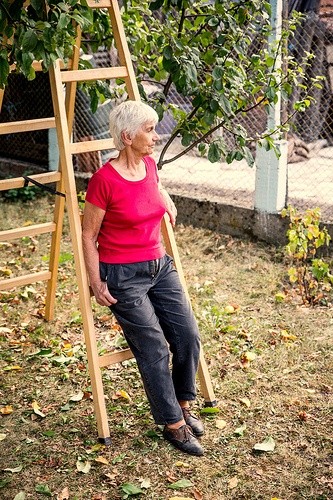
[0,1,218,448]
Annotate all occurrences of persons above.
[80,99,219,457]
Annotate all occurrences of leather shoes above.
[162,424,205,455]
[183,407,204,435]
